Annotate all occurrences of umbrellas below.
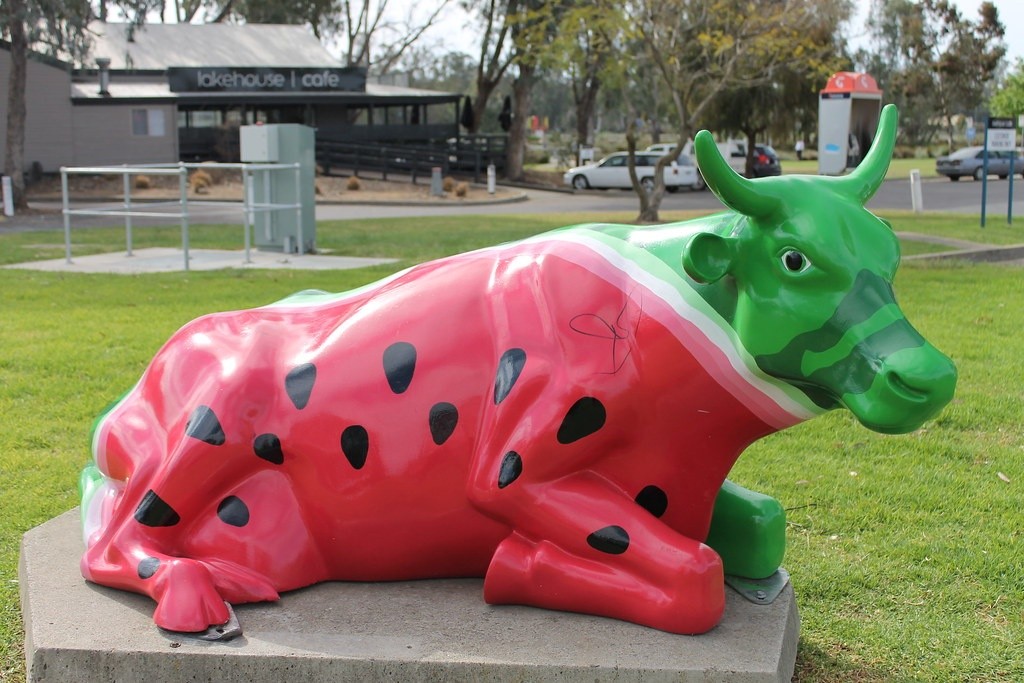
[496,96,512,133]
[459,95,476,135]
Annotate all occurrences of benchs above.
[315,131,507,185]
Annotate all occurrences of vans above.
[644,142,748,192]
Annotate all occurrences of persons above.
[795,136,805,161]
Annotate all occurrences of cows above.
[77,101,962,632]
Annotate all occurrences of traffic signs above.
[986,117,1017,151]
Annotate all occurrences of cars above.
[562,150,698,194]
[736,143,779,179]
[936,145,1024,181]
